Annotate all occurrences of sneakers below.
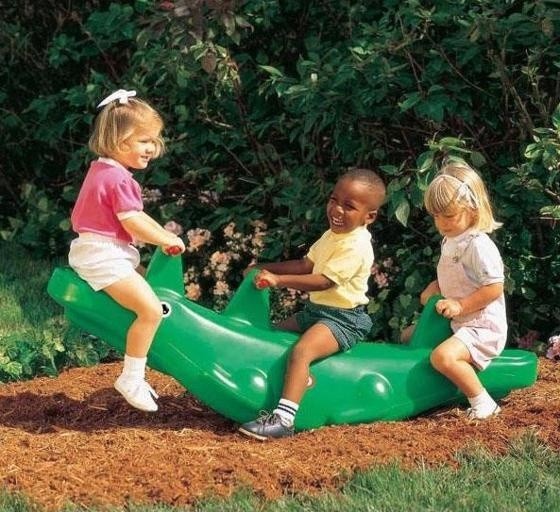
[237,414,295,441]
[465,401,501,421]
[113,375,159,412]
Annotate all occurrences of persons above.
[239,168,385,442]
[67,90,186,412]
[420,162,509,419]
